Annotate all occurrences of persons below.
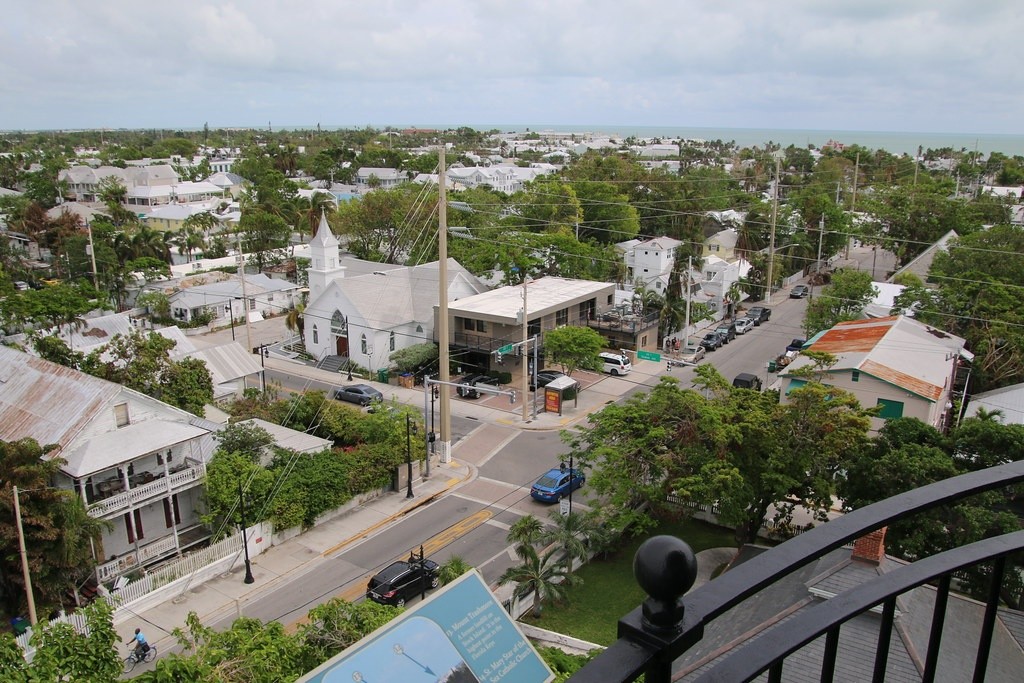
[125,628,149,663]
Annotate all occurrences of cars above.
[532,368,580,394]
[334,384,384,405]
[15,281,29,291]
[808,273,831,286]
[790,285,808,299]
[734,318,754,335]
[360,403,400,416]
[680,345,706,364]
[40,277,62,287]
[531,468,586,505]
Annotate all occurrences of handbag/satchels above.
[142,644,150,653]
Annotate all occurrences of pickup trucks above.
[744,306,772,326]
[786,339,807,353]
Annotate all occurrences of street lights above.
[406,421,417,499]
[431,386,439,452]
[408,551,424,600]
[816,230,837,273]
[560,458,572,516]
[341,323,352,381]
[225,306,235,341]
[765,244,799,303]
[261,347,270,392]
[239,491,255,584]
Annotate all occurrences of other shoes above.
[141,655,147,660]
[135,660,140,663]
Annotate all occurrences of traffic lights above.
[495,351,501,363]
[667,361,671,372]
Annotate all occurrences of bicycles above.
[120,644,157,674]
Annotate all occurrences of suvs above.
[731,373,763,396]
[714,323,736,344]
[366,556,443,607]
[456,372,501,399]
[699,331,723,352]
[588,351,632,376]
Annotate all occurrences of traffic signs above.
[637,350,660,362]
[499,344,513,355]
